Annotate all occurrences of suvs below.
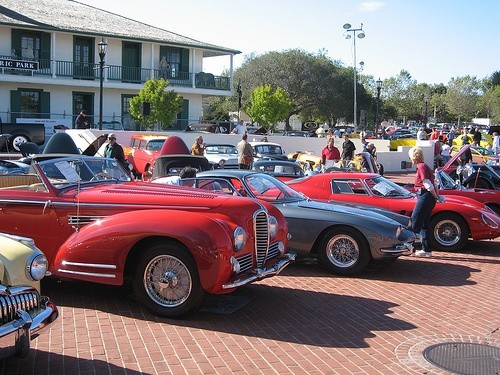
[94,119,124,131]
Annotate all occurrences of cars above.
[364,122,453,140]
[184,122,319,138]
[449,133,500,164]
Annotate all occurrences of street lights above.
[375,77,383,134]
[424,95,430,124]
[92,38,110,130]
[343,22,365,134]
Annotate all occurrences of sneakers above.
[414,249,432,257]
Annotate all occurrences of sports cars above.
[261,143,500,252]
[0,133,384,176]
[178,168,424,276]
[0,157,298,360]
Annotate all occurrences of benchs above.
[0,173,40,188]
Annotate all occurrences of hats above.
[108,133,116,138]
[82,110,87,115]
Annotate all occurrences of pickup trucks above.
[0,116,45,152]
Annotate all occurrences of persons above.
[150,119,499,257]
[101,133,126,181]
[76,110,88,129]
